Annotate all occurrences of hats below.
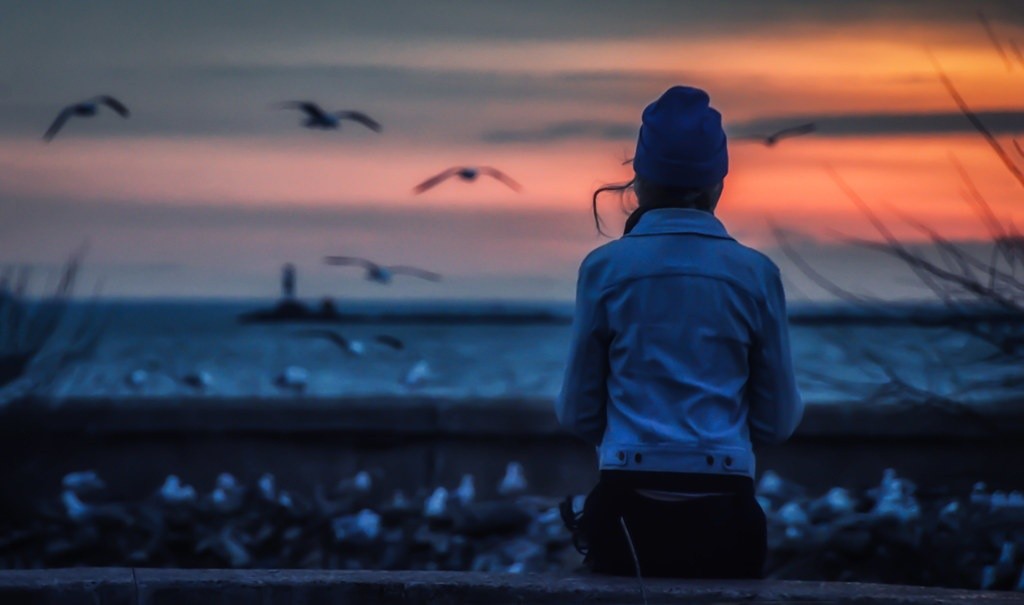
[633,86,727,190]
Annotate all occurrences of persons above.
[557,85,803,577]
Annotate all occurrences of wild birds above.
[39,92,129,138]
[36,257,1024,588]
[267,99,385,137]
[413,165,528,194]
